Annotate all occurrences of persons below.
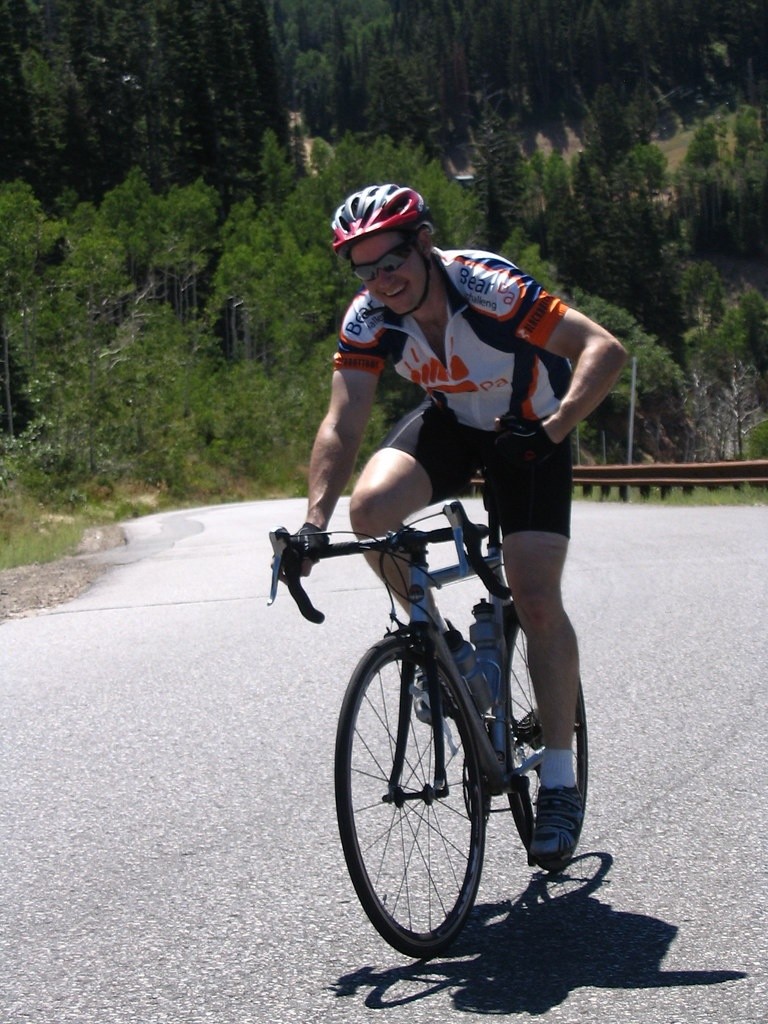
[271,185,628,864]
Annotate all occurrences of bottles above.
[443,629,492,714]
[466,597,500,697]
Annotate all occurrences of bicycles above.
[264,465,590,964]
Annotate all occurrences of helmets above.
[330,184,430,256]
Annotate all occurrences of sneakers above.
[530,785,583,859]
[416,667,452,718]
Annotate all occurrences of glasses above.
[351,224,426,280]
